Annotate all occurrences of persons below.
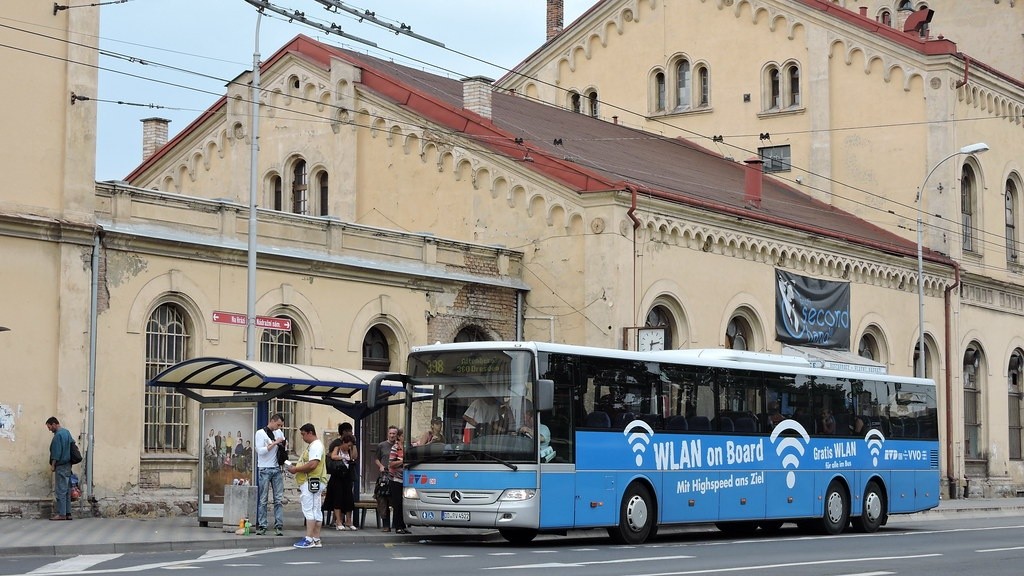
[817,409,835,435]
[768,401,787,432]
[374,427,413,534]
[518,410,550,462]
[286,423,326,548]
[418,417,446,446]
[205,429,251,474]
[327,422,359,531]
[462,398,506,444]
[855,404,884,438]
[46,416,78,520]
[255,414,288,536]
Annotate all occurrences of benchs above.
[304,499,393,530]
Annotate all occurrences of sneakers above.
[256,528,267,535]
[313,539,322,547]
[274,528,283,536]
[293,538,315,548]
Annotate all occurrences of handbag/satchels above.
[374,484,391,496]
[307,477,320,492]
[326,454,343,474]
[70,441,83,464]
[277,444,288,465]
[70,471,81,502]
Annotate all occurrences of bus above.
[366,340,942,545]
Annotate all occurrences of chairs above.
[542,405,920,442]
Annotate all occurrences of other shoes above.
[383,527,391,532]
[396,530,412,534]
[66,514,72,519]
[49,515,66,520]
[328,520,336,529]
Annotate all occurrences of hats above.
[431,417,444,423]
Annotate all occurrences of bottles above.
[239,517,244,529]
[244,519,250,535]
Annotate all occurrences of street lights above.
[911,141,991,378]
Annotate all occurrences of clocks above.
[638,325,668,352]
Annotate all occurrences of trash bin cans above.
[222,485,258,533]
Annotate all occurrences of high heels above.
[335,523,345,531]
[345,525,357,531]
